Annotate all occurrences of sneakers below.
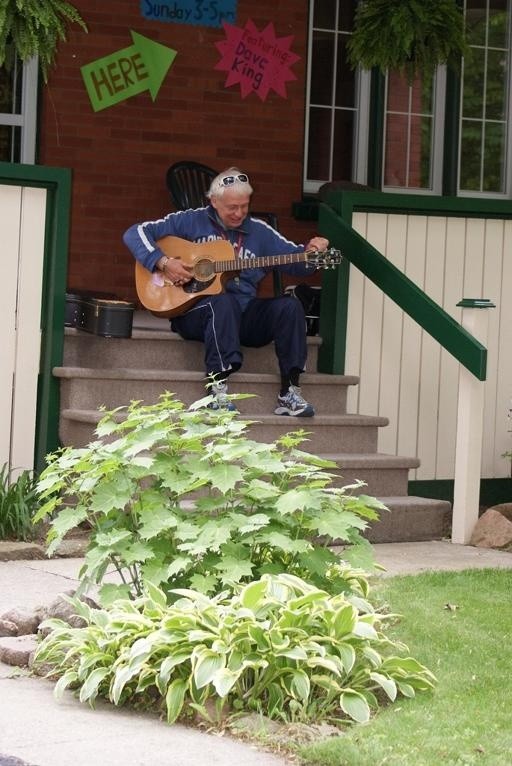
[275,385,314,417]
[207,384,237,411]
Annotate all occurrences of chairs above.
[165,161,283,297]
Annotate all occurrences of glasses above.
[220,174,249,186]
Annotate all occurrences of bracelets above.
[162,258,168,272]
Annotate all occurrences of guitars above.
[134,232,342,317]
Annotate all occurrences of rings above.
[177,280,180,283]
[174,282,176,285]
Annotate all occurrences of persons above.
[121,166,330,417]
